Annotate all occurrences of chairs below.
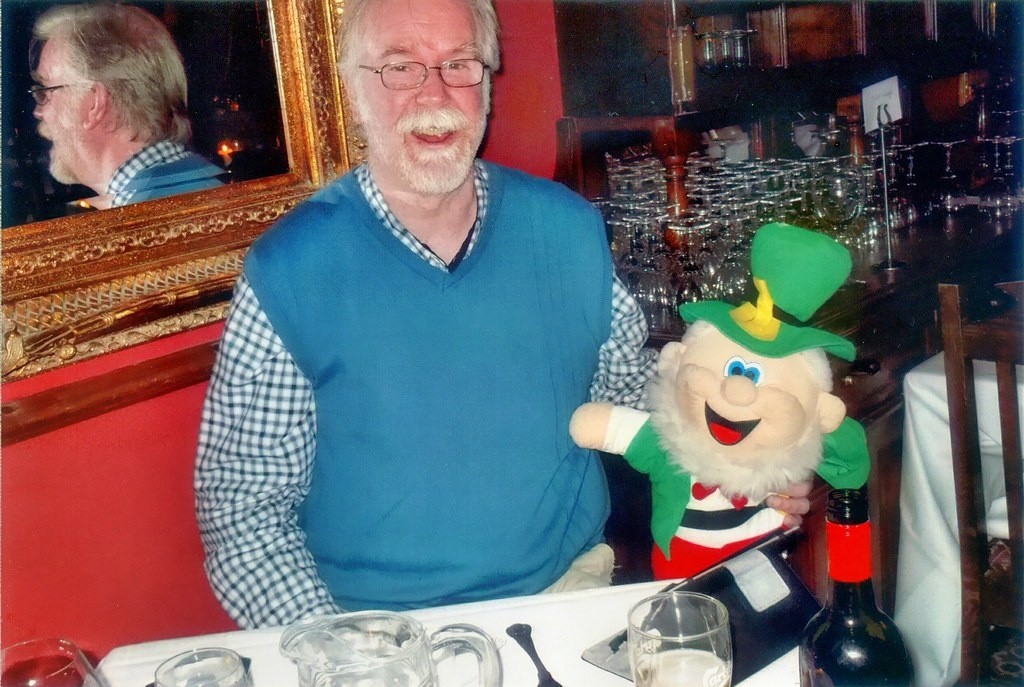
[938,286,1024,687]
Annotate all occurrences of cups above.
[282,610,503,687]
[0,638,102,687]
[145,648,255,686]
[627,592,733,687]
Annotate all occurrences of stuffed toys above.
[570,224,871,582]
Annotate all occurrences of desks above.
[84,575,809,687]
[895,347,1024,686]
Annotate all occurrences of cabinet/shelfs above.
[553,0,1024,262]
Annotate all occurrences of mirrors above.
[2,0,370,385]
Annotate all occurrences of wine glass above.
[589,27,1023,329]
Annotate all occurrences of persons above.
[192,1,813,630]
[34,0,235,210]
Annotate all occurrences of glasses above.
[357,50,491,91]
[25,79,81,105]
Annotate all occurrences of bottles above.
[799,484,913,686]
